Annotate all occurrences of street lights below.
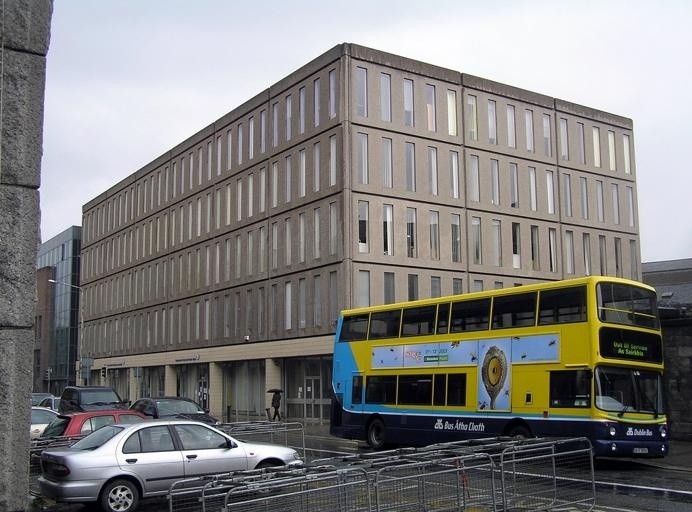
[47,278,85,386]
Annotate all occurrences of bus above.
[328,277,672,466]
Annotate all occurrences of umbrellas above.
[266,388,284,393]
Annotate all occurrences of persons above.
[272,391,281,421]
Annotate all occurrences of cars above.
[36,397,61,412]
[32,387,304,512]
